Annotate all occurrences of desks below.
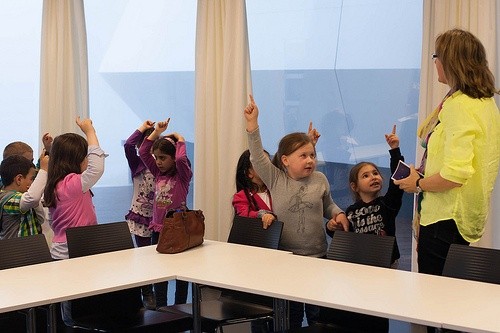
[0,240,500,333]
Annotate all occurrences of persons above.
[393,28,500,333]
[244,93,350,328]
[0,132,53,240]
[32,116,110,327]
[232,121,321,308]
[325,122,405,333]
[124,117,193,308]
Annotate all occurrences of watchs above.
[416,178,424,192]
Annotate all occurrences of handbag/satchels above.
[156,206,205,254]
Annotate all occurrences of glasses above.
[432,53,438,59]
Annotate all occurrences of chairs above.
[0,216,500,333]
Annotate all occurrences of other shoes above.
[143,296,154,309]
